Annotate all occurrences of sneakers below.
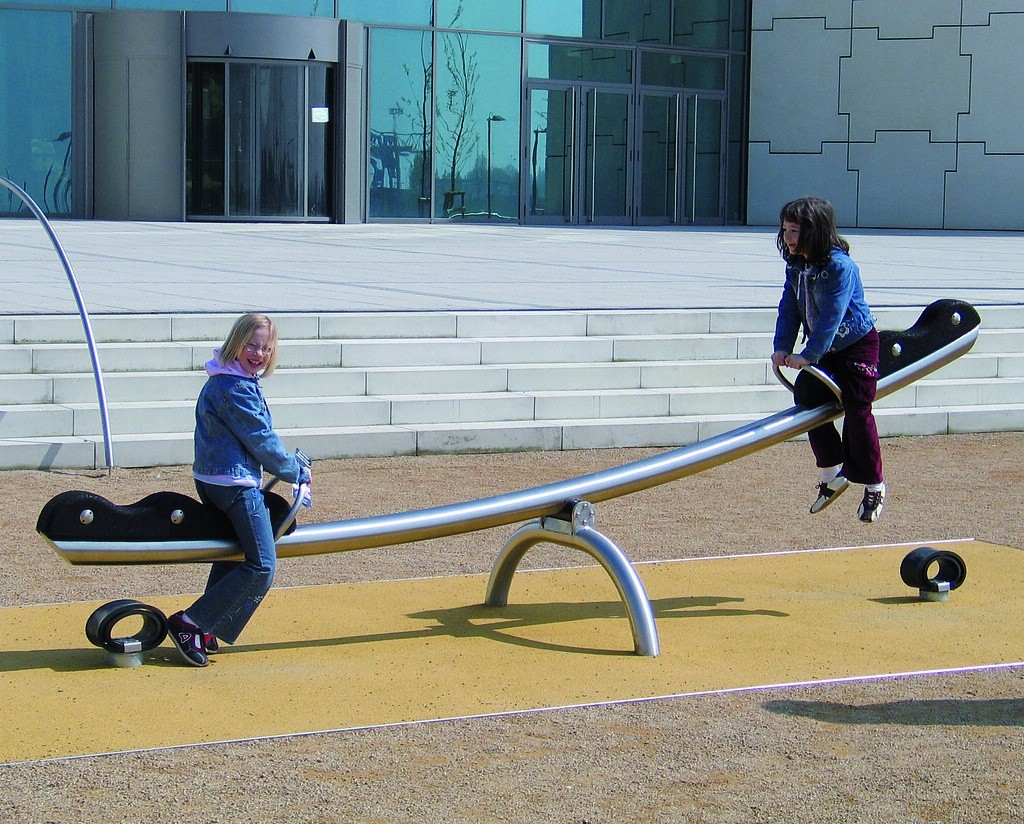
[809,472,849,513]
[203,633,218,654]
[167,612,210,667]
[858,487,886,525]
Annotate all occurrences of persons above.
[766,197,886,524]
[160,311,312,667]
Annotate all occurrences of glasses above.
[242,341,273,355]
